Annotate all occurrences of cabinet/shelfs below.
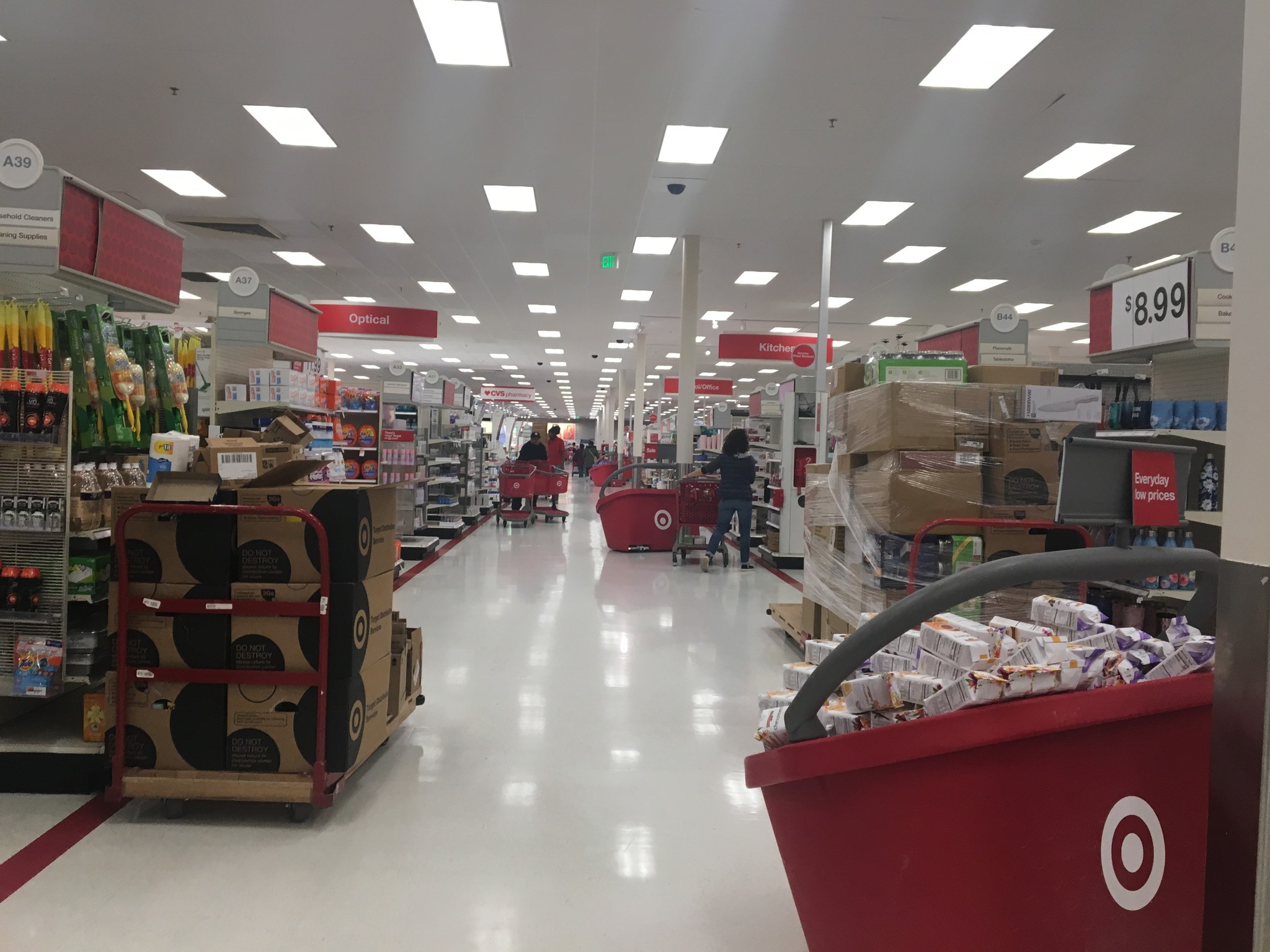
[748,392,816,571]
[1085,337,1229,603]
[172,320,500,581]
[692,415,768,547]
[0,527,110,794]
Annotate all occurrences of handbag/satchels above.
[594,456,597,463]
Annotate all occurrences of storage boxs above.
[801,359,1097,654]
[99,367,423,784]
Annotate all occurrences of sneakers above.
[741,565,754,569]
[700,555,711,572]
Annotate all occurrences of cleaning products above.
[297,412,346,483]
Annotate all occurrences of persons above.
[572,442,577,453]
[582,441,599,482]
[511,432,548,519]
[547,425,567,504]
[575,444,585,477]
[682,428,755,572]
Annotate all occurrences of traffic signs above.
[599,251,621,271]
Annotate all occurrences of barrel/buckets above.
[742,547,1219,952]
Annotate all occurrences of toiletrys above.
[485,466,500,474]
[488,491,500,501]
[462,427,497,449]
[481,476,499,488]
[489,453,499,462]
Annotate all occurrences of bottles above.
[410,450,415,465]
[19,461,147,531]
[395,473,400,488]
[387,449,392,465]
[405,449,410,466]
[409,473,414,489]
[382,448,387,462]
[390,473,394,484]
[400,449,405,465]
[393,449,398,465]
[404,473,409,489]
[382,472,388,484]
[1087,524,1197,590]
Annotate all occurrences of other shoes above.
[549,499,553,502]
[555,500,559,504]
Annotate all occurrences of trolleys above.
[105,501,425,823]
[571,461,586,478]
[767,518,1092,640]
[671,477,729,568]
[497,460,570,528]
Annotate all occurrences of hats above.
[531,432,541,437]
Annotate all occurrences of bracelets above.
[686,474,689,478]
